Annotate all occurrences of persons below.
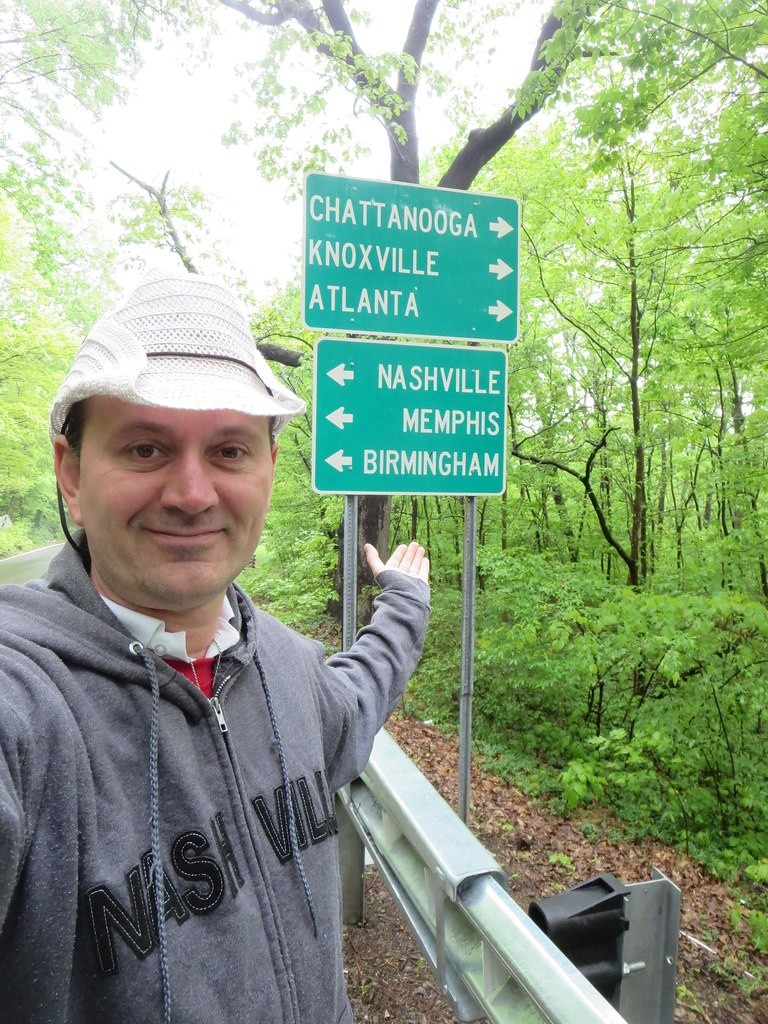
[0,274,428,1024]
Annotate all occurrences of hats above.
[48,270,307,440]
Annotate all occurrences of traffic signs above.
[304,172,522,344]
[311,337,509,497]
